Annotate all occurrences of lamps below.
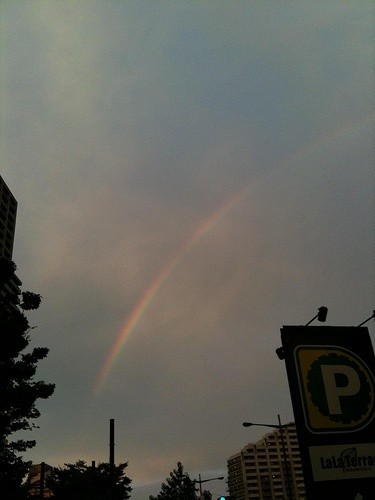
[305,305,329,326]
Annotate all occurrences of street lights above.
[182,471,224,499]
[242,415,300,499]
